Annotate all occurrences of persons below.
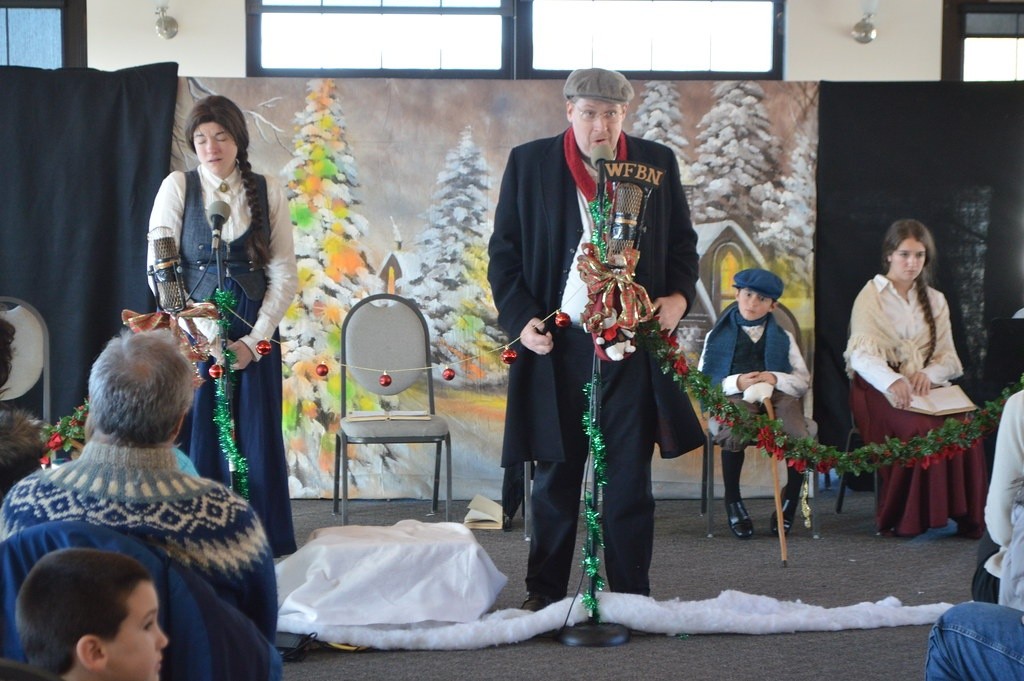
[696,268,811,539]
[488,69,701,612]
[0,548,168,681]
[0,331,277,643]
[0,403,44,502]
[145,94,296,557]
[842,218,990,536]
[923,390,1024,681]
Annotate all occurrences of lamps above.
[154,1,178,39]
[851,0,878,42]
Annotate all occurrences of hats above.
[563,68,634,104]
[732,268,784,302]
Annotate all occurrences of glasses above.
[573,101,623,122]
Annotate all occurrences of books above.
[885,385,977,416]
[347,410,432,422]
[464,495,503,529]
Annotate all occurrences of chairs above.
[701,299,821,540]
[0,296,50,423]
[333,293,452,527]
[1,521,281,681]
[835,322,884,536]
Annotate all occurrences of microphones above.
[147,225,185,310]
[207,201,231,250]
[590,145,614,209]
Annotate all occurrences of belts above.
[569,321,591,333]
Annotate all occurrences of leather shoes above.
[724,497,753,538]
[770,487,798,536]
[521,595,552,611]
